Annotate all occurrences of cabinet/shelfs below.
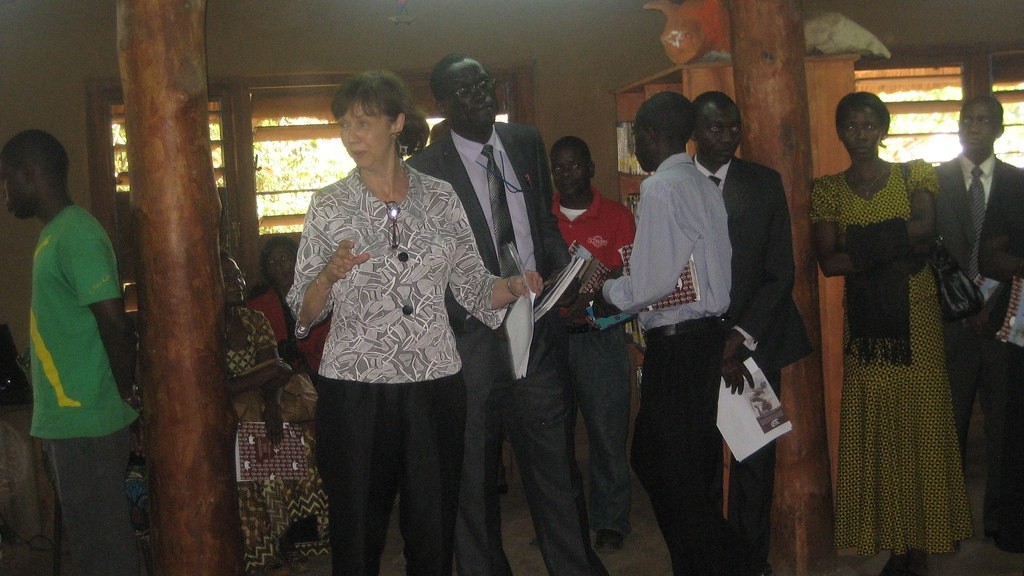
[608,51,861,576]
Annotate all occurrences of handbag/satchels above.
[994,259,1024,347]
[901,163,985,322]
[271,334,319,422]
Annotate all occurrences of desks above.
[0,401,69,549]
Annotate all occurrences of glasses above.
[220,271,248,286]
[384,200,401,249]
[445,77,497,100]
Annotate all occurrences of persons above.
[285,70,543,576]
[692,92,814,563]
[0,129,147,576]
[218,235,332,576]
[595,90,774,576]
[924,95,1024,555]
[807,91,974,576]
[404,54,609,576]
[549,137,635,554]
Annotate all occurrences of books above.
[718,357,792,462]
[236,422,309,481]
[569,241,639,331]
[615,121,656,176]
[534,258,587,322]
[620,242,701,312]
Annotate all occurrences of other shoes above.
[291,515,320,548]
[878,548,927,576]
[985,517,1024,553]
[594,528,624,552]
[496,462,508,494]
[529,536,538,546]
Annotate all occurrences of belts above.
[566,321,588,335]
[643,316,726,342]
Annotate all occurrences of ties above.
[967,164,985,282]
[481,144,521,278]
[708,175,721,187]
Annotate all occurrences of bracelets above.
[507,277,520,297]
[316,271,332,293]
